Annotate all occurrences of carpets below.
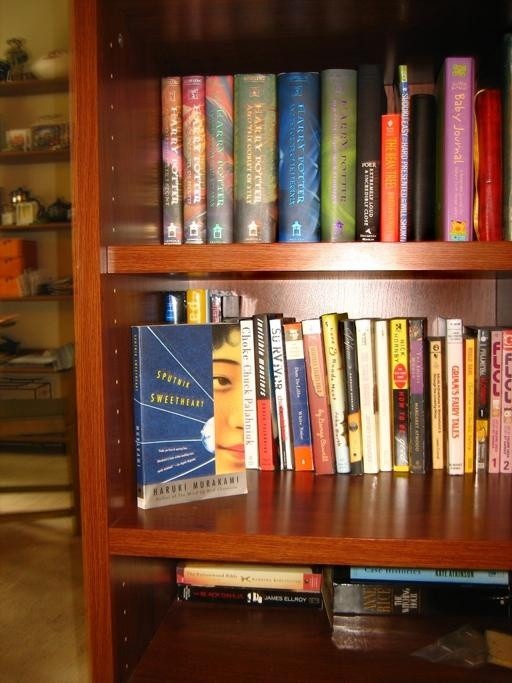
[0,449,87,682]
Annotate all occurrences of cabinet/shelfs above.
[0,0,81,534]
[69,0,512,682]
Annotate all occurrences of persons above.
[210,324,245,475]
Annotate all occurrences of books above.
[162,291,243,322]
[382,114,398,240]
[174,564,322,589]
[172,588,325,608]
[0,345,81,534]
[279,72,321,243]
[333,584,465,616]
[354,64,383,241]
[207,77,233,244]
[485,623,511,669]
[242,312,512,473]
[397,66,409,243]
[162,78,183,243]
[321,69,356,241]
[236,75,278,243]
[183,76,207,244]
[409,93,439,241]
[349,567,510,583]
[478,88,501,243]
[442,56,476,241]
[132,324,248,510]
